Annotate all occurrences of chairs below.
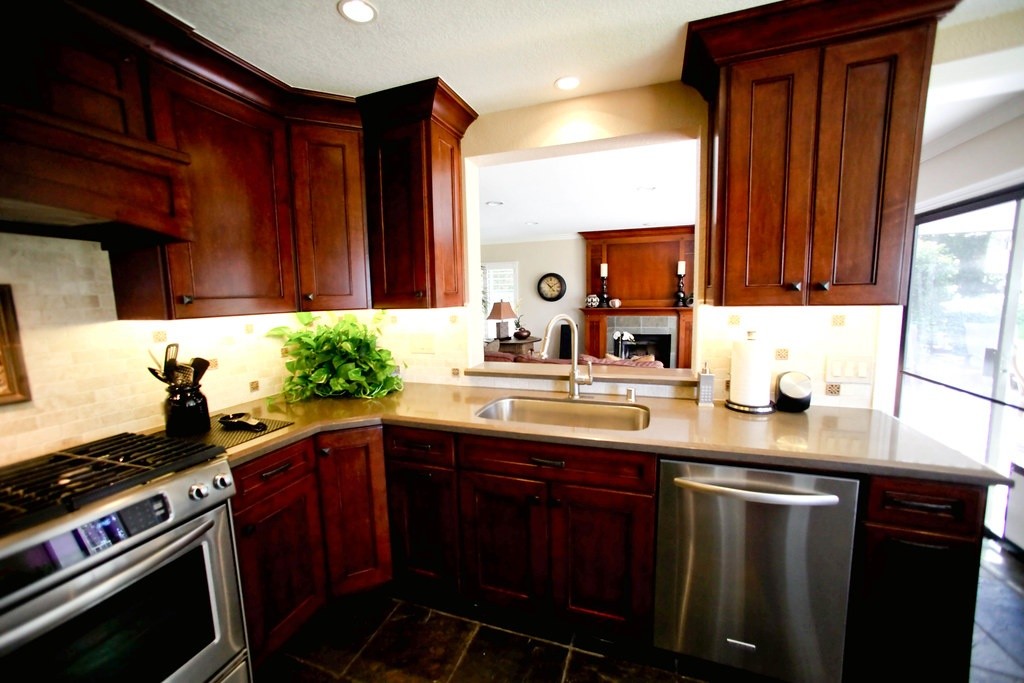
[484,339,500,352]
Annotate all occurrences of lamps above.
[486,299,518,339]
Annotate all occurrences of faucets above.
[538,313,593,400]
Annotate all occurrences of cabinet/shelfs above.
[845,476,988,683]
[680,0,961,307]
[0,0,479,321]
[226,424,658,676]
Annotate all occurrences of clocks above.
[537,273,566,302]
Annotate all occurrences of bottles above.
[164,384,210,437]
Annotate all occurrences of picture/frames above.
[0,284,32,406]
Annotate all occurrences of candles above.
[677,260,686,275]
[600,262,608,277]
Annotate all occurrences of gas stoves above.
[0,431,237,604]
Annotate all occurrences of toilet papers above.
[730,339,773,406]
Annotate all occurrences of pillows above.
[630,354,655,363]
[604,353,621,361]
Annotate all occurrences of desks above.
[484,336,542,355]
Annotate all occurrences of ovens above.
[1,499,254,683]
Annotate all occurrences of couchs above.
[484,351,664,369]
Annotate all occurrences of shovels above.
[164,343,179,374]
[191,358,210,385]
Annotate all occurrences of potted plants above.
[514,316,522,332]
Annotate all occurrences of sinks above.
[475,395,650,431]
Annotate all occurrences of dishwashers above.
[646,459,861,683]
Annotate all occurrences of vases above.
[609,299,622,309]
[584,294,600,308]
[514,328,530,340]
